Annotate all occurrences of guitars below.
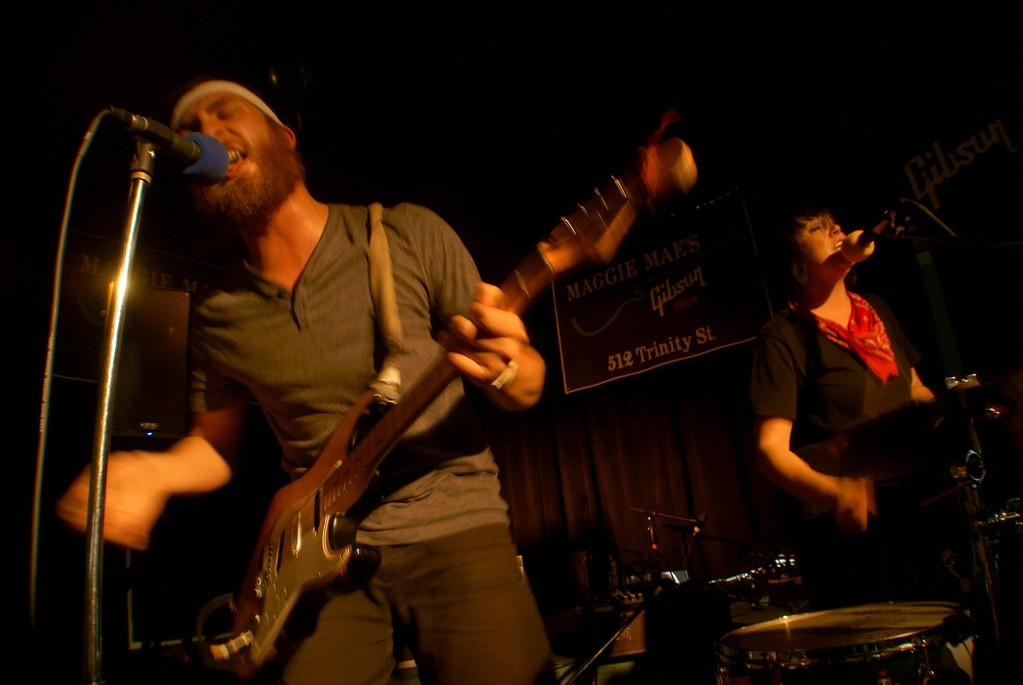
[232,136,697,683]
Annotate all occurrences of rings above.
[491,363,517,389]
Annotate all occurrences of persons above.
[60,83,556,685]
[747,198,946,603]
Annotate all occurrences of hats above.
[172,81,284,134]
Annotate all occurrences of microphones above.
[841,198,904,262]
[691,512,708,538]
[105,106,228,188]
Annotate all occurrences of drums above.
[718,603,974,684]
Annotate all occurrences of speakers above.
[104,281,192,440]
